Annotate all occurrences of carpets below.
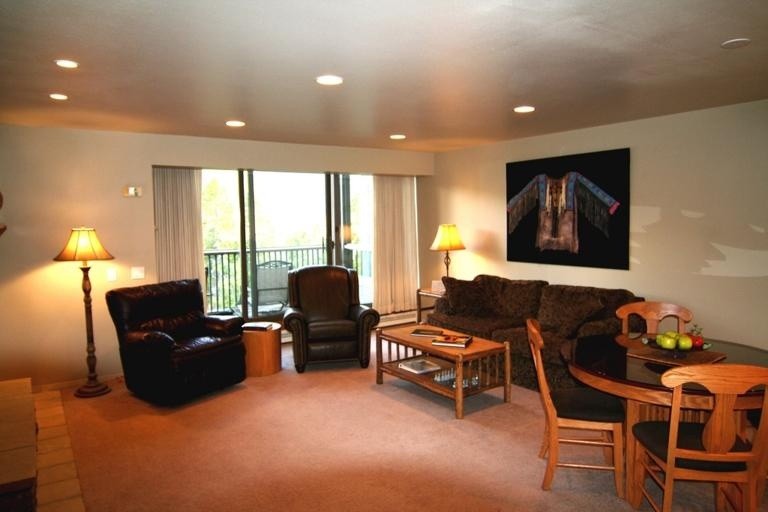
[59,322,768,511]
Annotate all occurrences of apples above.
[656,330,704,350]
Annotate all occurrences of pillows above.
[441,275,632,340]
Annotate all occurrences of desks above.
[567,332,767,503]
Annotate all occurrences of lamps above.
[52,227,115,398]
[428,224,466,278]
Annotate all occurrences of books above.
[398,358,442,375]
[432,335,473,348]
[241,322,272,330]
[410,329,442,337]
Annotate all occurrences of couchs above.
[426,274,647,395]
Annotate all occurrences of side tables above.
[416,285,446,325]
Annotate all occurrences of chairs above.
[281,264,380,374]
[104,277,248,409]
[631,362,768,512]
[526,317,625,499]
[615,300,693,335]
